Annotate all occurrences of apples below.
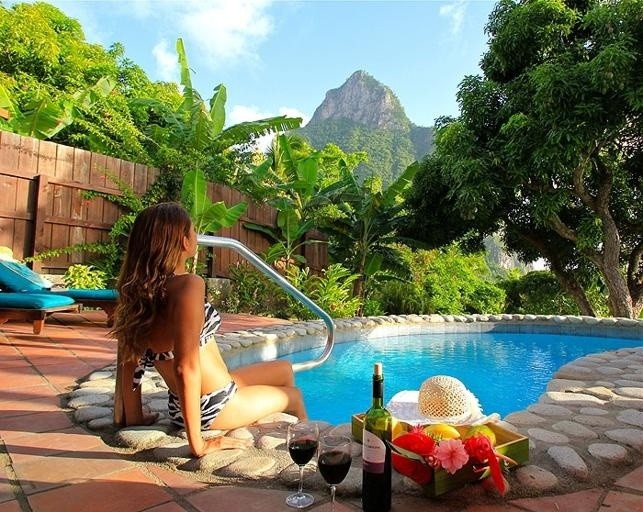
[392,422,413,441]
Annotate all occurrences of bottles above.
[364,362,392,512]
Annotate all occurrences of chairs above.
[0,292,75,335]
[0,259,121,328]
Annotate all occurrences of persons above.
[107,200,308,458]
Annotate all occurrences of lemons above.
[423,422,460,444]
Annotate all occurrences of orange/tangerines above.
[464,426,496,446]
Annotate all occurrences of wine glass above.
[320,434,353,512]
[287,424,319,507]
